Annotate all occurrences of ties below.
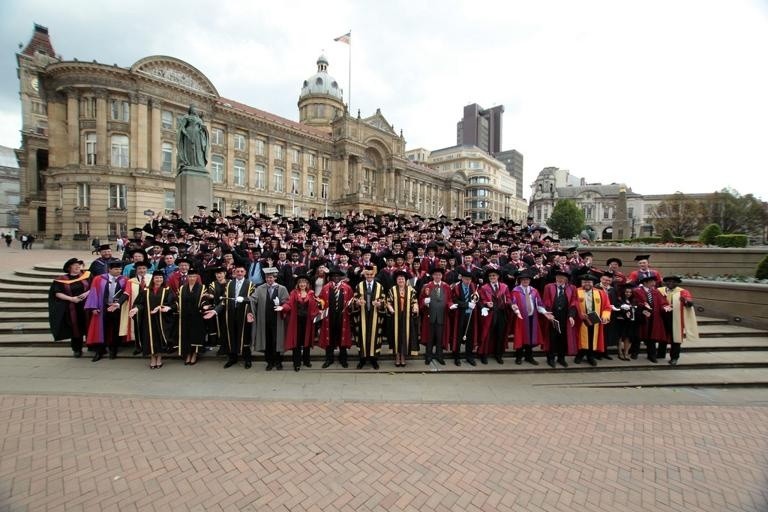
[495,284,498,291]
[108,277,116,305]
[367,283,371,311]
[558,285,564,309]
[525,288,531,314]
[465,287,469,302]
[333,284,338,312]
[648,289,652,303]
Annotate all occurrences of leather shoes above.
[150,347,379,371]
[395,354,613,367]
[619,351,677,365]
[74,348,149,361]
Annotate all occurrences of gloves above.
[621,304,630,310]
[611,305,621,311]
[236,296,244,302]
[450,304,459,309]
[469,302,476,309]
[273,296,279,306]
[481,308,490,317]
[424,297,430,303]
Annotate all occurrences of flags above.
[334,32,350,44]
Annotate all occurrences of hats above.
[663,276,682,282]
[620,284,636,289]
[127,210,599,281]
[640,276,658,283]
[97,244,112,251]
[606,258,622,267]
[634,255,650,261]
[600,270,614,277]
[63,259,83,272]
[106,260,129,268]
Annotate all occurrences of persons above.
[175,105,209,176]
[20,234,34,249]
[5,232,12,247]
[50,204,700,372]
